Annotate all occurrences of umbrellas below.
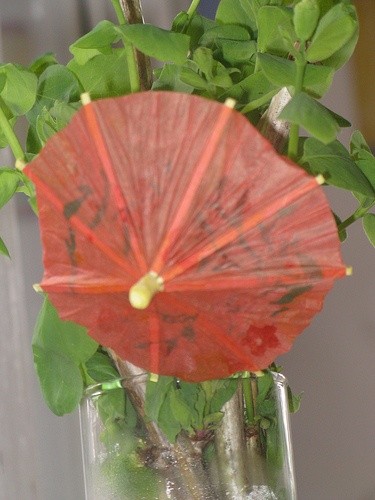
[14,89,352,383]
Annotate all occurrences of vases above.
[77,363,300,500]
